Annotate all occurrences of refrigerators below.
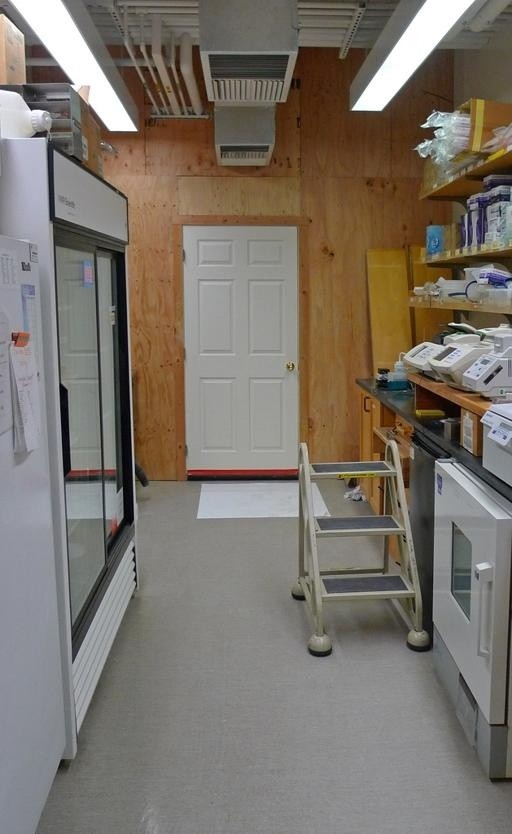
[0,137,140,761]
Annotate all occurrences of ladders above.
[291,441,431,656]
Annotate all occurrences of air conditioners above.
[197,1,301,103]
[211,102,276,170]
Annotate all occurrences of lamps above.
[345,0,474,112]
[9,0,144,136]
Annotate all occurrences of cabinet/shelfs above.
[355,385,394,518]
[408,145,512,445]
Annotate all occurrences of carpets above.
[65,481,118,519]
[197,482,329,518]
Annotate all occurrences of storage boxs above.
[448,98,512,163]
[457,407,483,456]
[385,489,410,567]
[35,93,103,179]
[0,12,27,86]
